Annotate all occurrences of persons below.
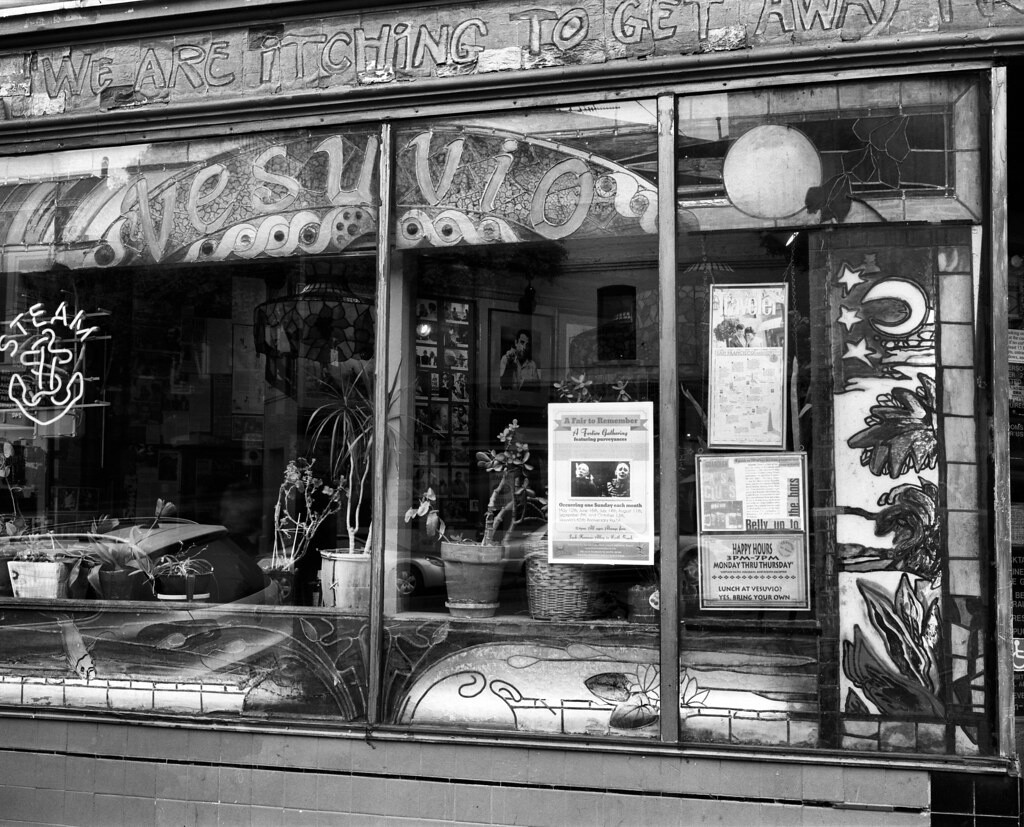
[602,463,630,496]
[500,329,540,390]
[571,463,597,497]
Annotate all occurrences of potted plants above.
[258,357,599,620]
[7,496,214,602]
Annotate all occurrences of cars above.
[138,432,279,499]
[502,506,699,590]
[253,533,446,601]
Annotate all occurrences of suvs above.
[0,517,295,678]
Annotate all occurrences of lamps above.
[509,271,537,315]
[414,321,433,341]
